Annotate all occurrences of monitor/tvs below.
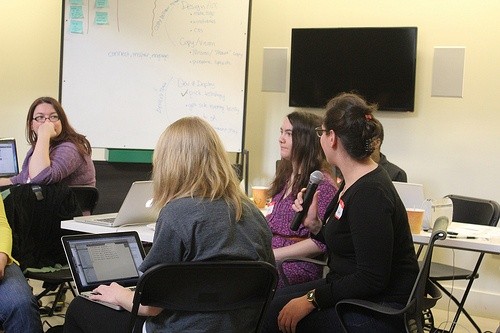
[0,138,19,178]
[289,25,416,112]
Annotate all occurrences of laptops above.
[61,232,146,310]
[393,181,432,232]
[72,180,157,228]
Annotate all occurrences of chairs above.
[25,192,500,333]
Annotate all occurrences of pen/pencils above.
[447,232,458,235]
[424,229,433,232]
[449,236,476,239]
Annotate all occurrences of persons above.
[0,97,96,216]
[0,194,45,333]
[47,116,276,333]
[371,119,407,182]
[262,90,420,333]
[265,112,339,288]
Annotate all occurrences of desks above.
[412,222,500,333]
[60,218,155,244]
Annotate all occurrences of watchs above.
[306,290,319,309]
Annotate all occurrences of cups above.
[405,209,424,234]
[251,187,270,209]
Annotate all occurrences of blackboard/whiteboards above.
[58,0,252,153]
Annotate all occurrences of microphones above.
[289,170,323,230]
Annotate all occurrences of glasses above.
[33,115,60,123]
[315,127,330,137]
